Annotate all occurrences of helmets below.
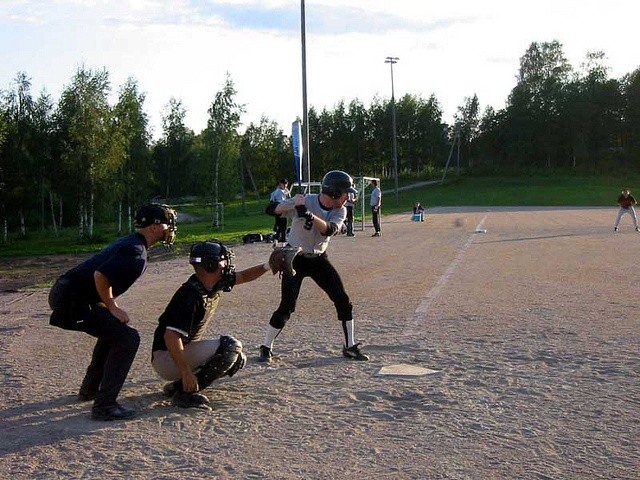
[322,170,358,199]
[133,204,178,245]
[189,241,236,292]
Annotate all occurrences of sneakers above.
[343,343,368,360]
[171,391,209,407]
[258,345,273,362]
[164,383,177,398]
[370,232,380,237]
[79,378,99,401]
[92,402,136,420]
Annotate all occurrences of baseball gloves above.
[269,247,303,277]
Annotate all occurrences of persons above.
[346,182,358,236]
[148,238,302,404]
[613,188,640,232]
[258,169,372,364]
[45,200,179,422]
[412,202,425,222]
[270,178,291,241]
[368,179,383,236]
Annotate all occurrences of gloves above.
[295,205,308,219]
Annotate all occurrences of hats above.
[280,179,288,184]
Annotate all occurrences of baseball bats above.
[292,120,305,220]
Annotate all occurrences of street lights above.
[384,57,400,201]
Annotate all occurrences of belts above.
[298,251,328,258]
[56,278,74,287]
